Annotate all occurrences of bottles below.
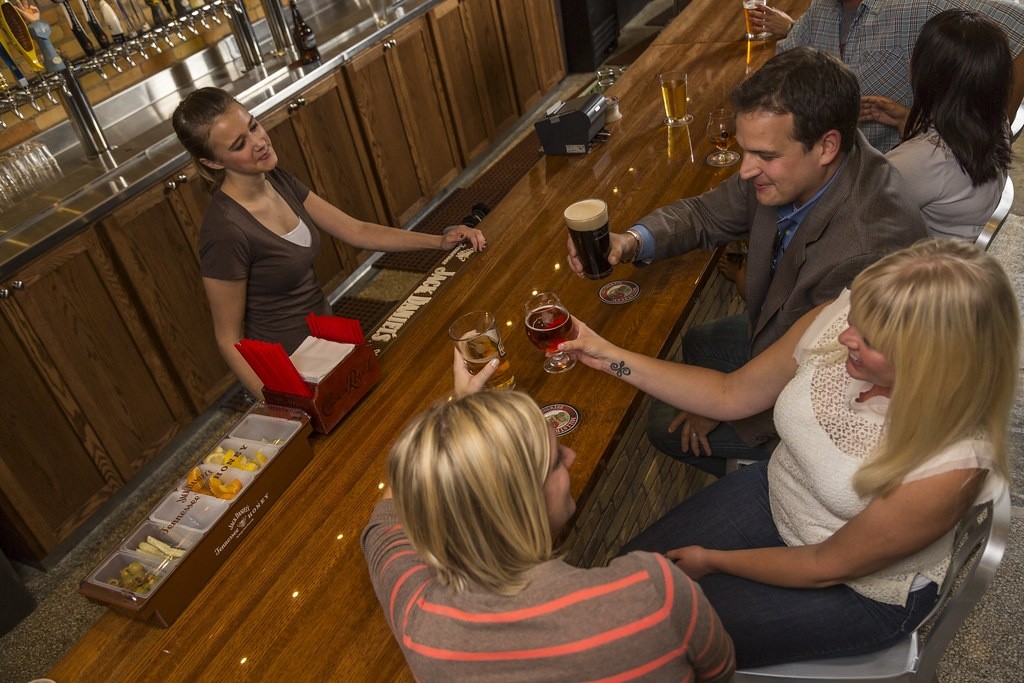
[288,0,322,66]
[602,94,623,123]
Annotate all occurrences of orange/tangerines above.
[188,466,243,501]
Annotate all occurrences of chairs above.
[1009,97,1024,145]
[972,175,1014,253]
[727,470,1010,683]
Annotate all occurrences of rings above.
[689,432,698,438]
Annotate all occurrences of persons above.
[860,10,1016,245]
[544,238,1024,683]
[747,1,796,35]
[567,46,933,479]
[14,0,40,23]
[776,0,1024,155]
[362,328,736,683]
[173,87,487,401]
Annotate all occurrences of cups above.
[745,39,769,75]
[658,71,688,122]
[667,122,693,168]
[595,65,629,98]
[741,0,767,38]
[447,309,516,391]
[563,199,612,280]
[0,143,58,203]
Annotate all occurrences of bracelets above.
[621,229,641,265]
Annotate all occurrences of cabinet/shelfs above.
[0,0,566,555]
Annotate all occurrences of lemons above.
[205,446,267,472]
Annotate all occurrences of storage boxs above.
[261,343,384,435]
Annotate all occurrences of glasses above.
[771,233,785,269]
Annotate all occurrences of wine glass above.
[525,291,578,373]
[706,109,738,164]
